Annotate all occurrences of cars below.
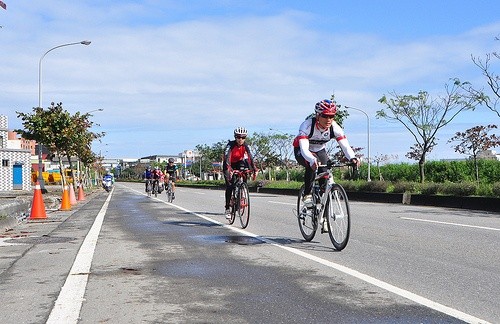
[155,180,164,191]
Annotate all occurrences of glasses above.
[319,114,338,118]
[235,135,247,139]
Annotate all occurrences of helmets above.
[168,158,175,162]
[234,127,248,137]
[314,99,337,116]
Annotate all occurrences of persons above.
[103,158,180,200]
[223,126,257,221]
[294,100,360,233]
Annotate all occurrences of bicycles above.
[145,179,154,198]
[228,163,252,229]
[166,176,175,203]
[154,179,161,198]
[292,159,357,251]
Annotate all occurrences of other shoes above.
[301,189,315,207]
[225,208,231,219]
[322,220,333,232]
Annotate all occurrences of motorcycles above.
[102,176,112,193]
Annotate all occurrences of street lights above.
[345,106,371,182]
[76,108,104,183]
[37,39,91,194]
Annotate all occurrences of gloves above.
[252,168,259,181]
[226,169,234,182]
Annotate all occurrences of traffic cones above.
[58,184,72,211]
[69,183,78,205]
[76,184,85,201]
[27,179,48,219]
